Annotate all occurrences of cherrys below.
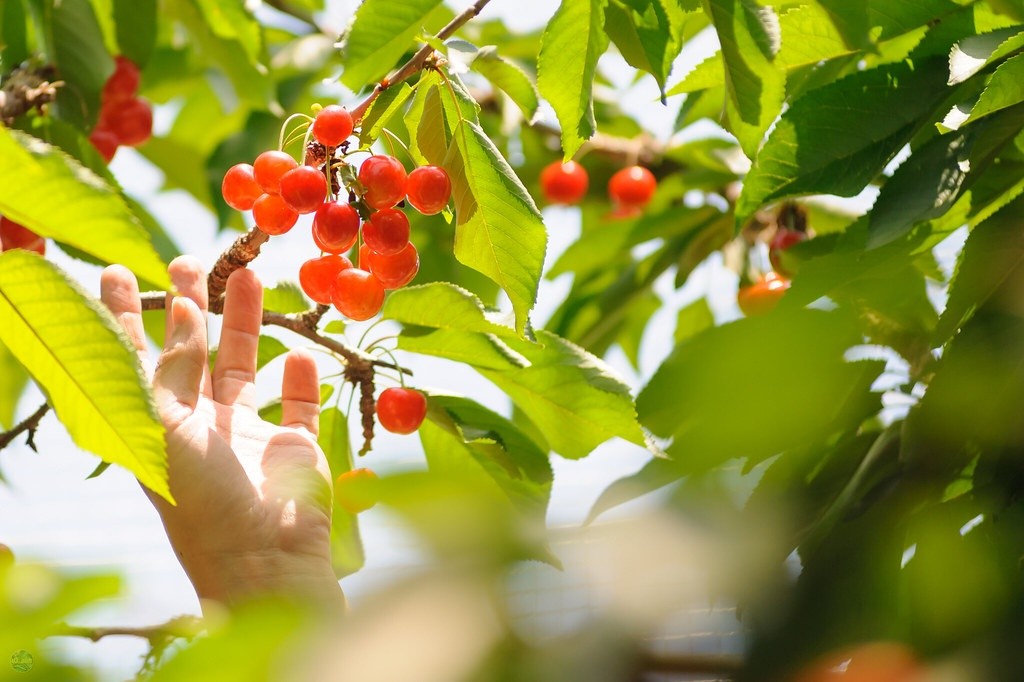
[0,55,151,257]
[222,105,809,435]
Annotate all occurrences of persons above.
[100,254,348,682]
